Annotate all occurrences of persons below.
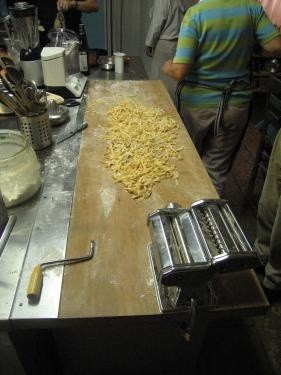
[6,0,99,52]
[253,0,281,307]
[144,0,199,105]
[163,0,281,200]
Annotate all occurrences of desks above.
[250,70,281,119]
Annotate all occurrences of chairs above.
[250,93,281,187]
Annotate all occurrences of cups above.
[113,52,126,73]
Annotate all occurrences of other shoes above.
[263,277,281,290]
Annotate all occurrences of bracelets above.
[75,0,78,10]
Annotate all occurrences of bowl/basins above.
[97,56,114,70]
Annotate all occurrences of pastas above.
[103,97,183,202]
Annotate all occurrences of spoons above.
[0,57,47,117]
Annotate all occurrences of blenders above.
[3,2,43,85]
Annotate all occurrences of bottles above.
[78,23,89,76]
[0,129,43,208]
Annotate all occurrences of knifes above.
[56,123,88,144]
